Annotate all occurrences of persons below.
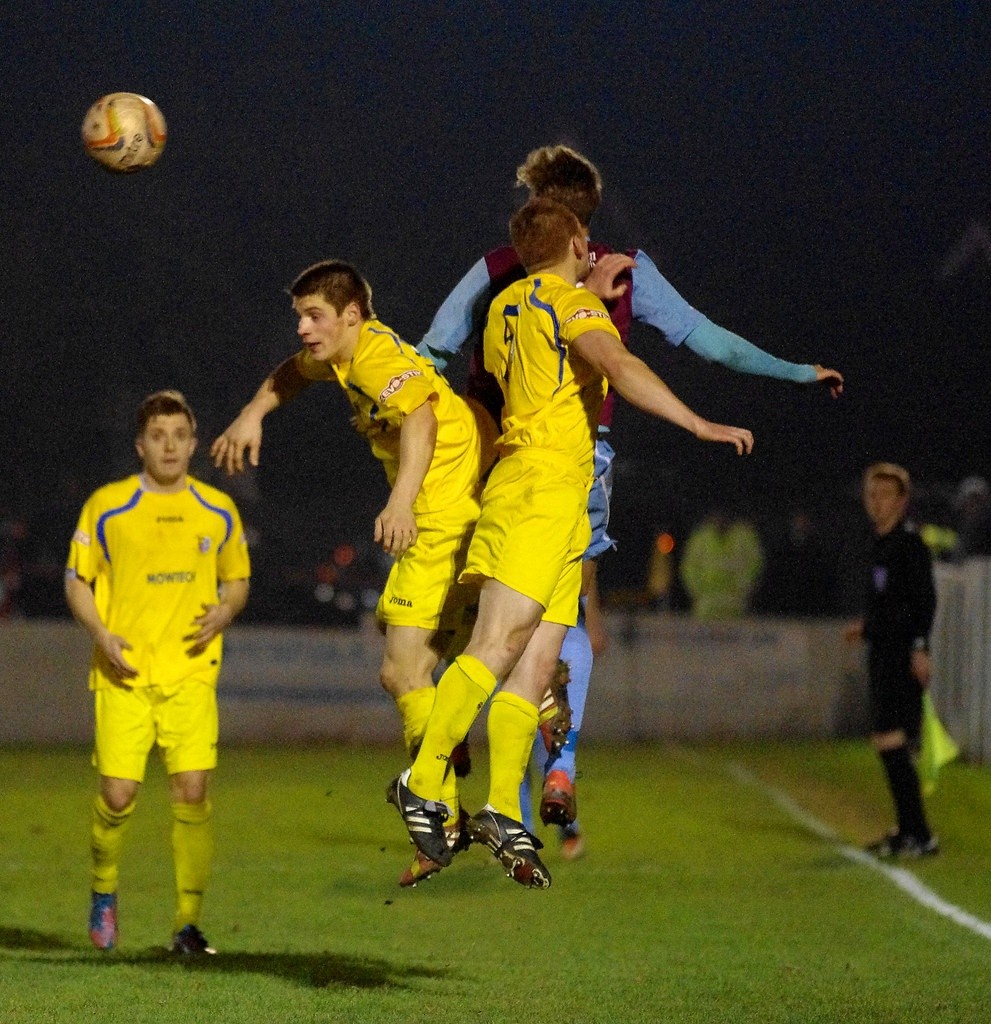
[388,201,752,886]
[415,144,841,827]
[66,393,250,956]
[360,477,991,859]
[210,260,573,889]
[847,463,942,859]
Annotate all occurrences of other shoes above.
[562,834,583,860]
[868,834,939,860]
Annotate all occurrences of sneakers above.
[398,808,469,888]
[90,891,117,950]
[169,924,207,954]
[540,770,577,826]
[454,735,472,778]
[467,809,551,890]
[387,768,452,867]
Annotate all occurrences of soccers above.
[80,92,168,173]
